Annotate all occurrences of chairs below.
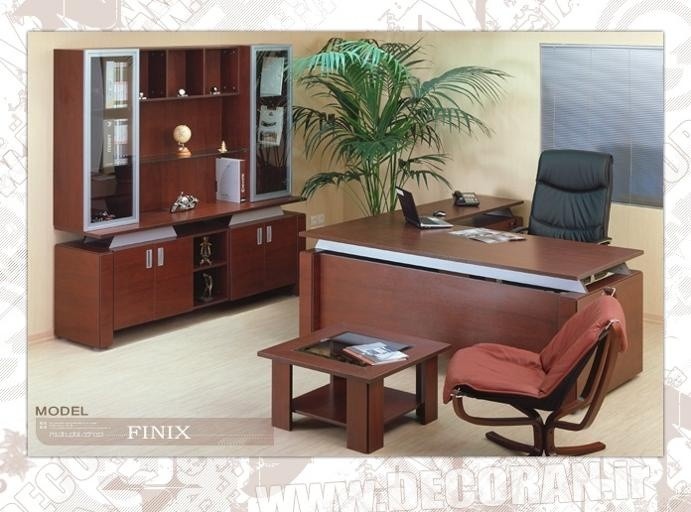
[441,288,629,457]
[511,149,618,246]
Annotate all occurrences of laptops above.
[394,185,454,229]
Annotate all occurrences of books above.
[257,55,287,98]
[341,348,409,366]
[257,104,284,147]
[102,59,129,111]
[346,341,409,362]
[102,118,133,169]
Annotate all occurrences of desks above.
[257,323,453,455]
[298,193,644,415]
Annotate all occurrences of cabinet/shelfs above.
[54,43,308,352]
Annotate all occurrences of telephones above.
[452,191,480,207]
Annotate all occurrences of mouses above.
[433,210,446,217]
